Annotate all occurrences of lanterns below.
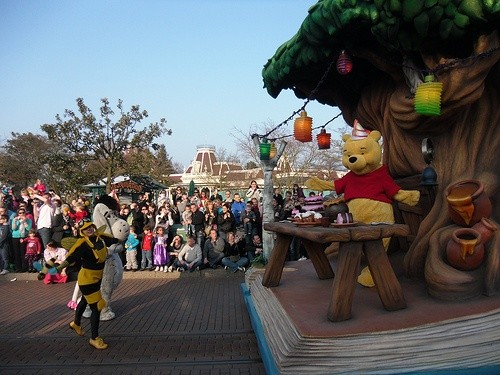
[336,49,352,75]
[413,70,443,117]
[259,138,271,160]
[269,139,277,159]
[317,125,331,149]
[293,112,313,142]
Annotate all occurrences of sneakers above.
[89,336,108,349]
[69,321,85,335]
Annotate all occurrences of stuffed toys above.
[67,193,130,321]
[306,130,421,286]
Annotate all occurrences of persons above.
[0,177,337,282]
[55,219,125,349]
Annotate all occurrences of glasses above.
[78,217,90,226]
[19,212,25,214]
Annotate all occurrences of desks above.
[262,220,409,322]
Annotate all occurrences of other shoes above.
[160,266,163,271]
[168,266,172,272]
[28,269,31,273]
[240,267,245,271]
[125,269,131,271]
[224,266,229,270]
[148,268,152,271]
[164,266,168,272]
[155,266,159,272]
[32,269,35,272]
[196,266,199,270]
[1,270,8,275]
[140,268,144,271]
[177,267,184,272]
[133,269,136,272]
